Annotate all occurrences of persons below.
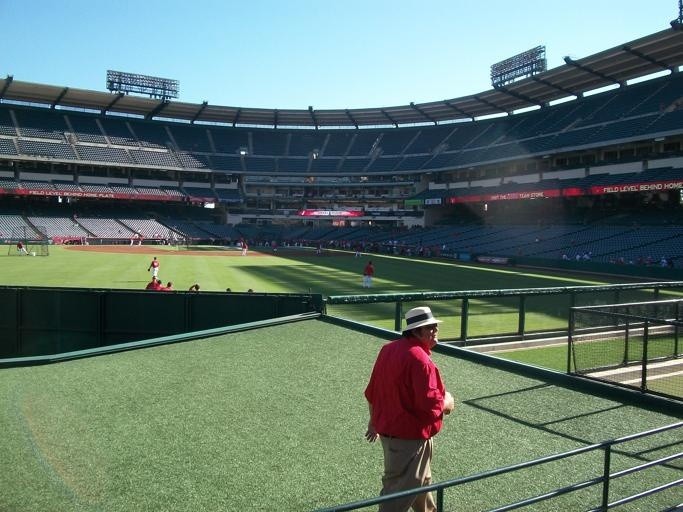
[148,257,160,277]
[315,239,448,259]
[17,241,29,255]
[130,234,145,247]
[146,276,174,290]
[237,236,308,256]
[562,252,593,260]
[189,284,200,292]
[363,307,454,512]
[611,252,672,269]
[363,261,373,288]
[155,234,174,246]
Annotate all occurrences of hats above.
[401,306,444,331]
